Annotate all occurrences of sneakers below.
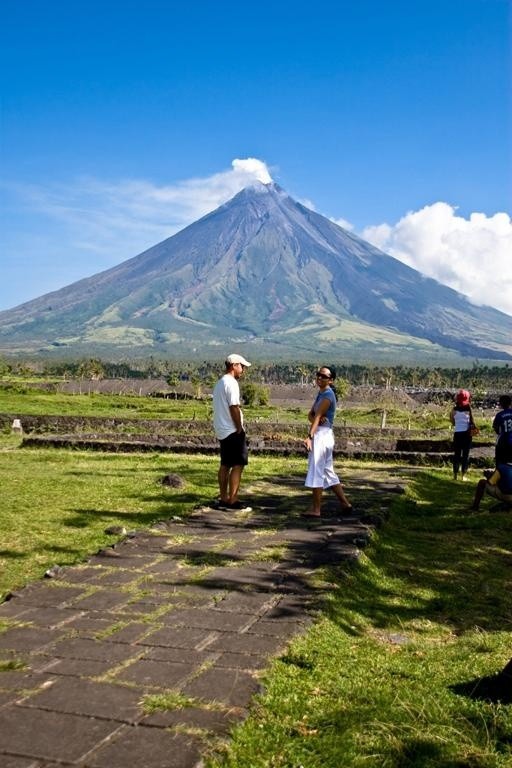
[218,499,253,512]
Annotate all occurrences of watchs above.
[308,433,314,439]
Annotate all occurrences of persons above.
[473,454,512,512]
[492,394,512,468]
[212,353,254,514]
[449,388,471,481]
[301,365,353,517]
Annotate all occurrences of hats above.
[226,354,251,367]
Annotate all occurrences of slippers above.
[335,506,353,512]
[301,511,319,518]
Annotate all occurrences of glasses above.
[316,371,331,380]
